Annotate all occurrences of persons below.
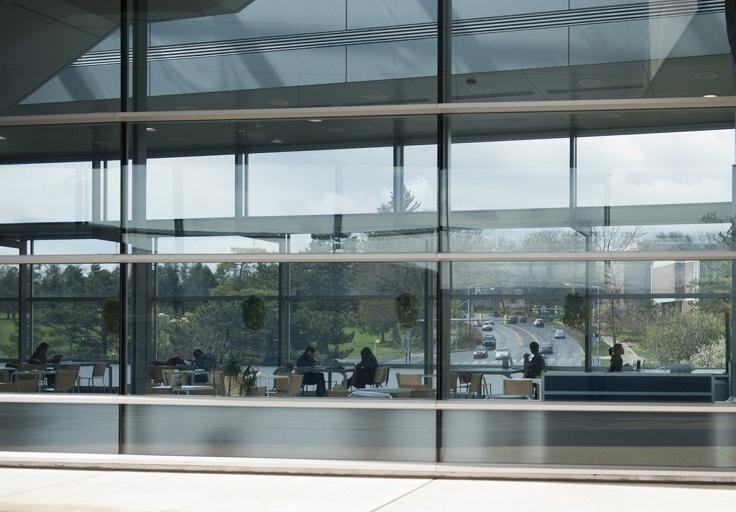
[609,344,624,372]
[342,347,378,390]
[187,349,218,394]
[297,346,326,397]
[523,342,545,378]
[31,342,55,387]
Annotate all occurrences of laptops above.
[510,359,522,369]
[44,356,62,363]
[631,358,645,371]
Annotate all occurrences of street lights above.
[564,282,600,357]
[468,283,483,351]
[470,288,496,335]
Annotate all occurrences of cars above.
[540,342,553,354]
[533,319,544,327]
[474,345,488,359]
[482,321,492,330]
[495,347,511,359]
[509,314,526,324]
[482,334,496,347]
[554,329,564,338]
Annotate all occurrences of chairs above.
[503,380,534,396]
[391,372,435,398]
[373,368,389,388]
[154,365,304,397]
[324,386,350,396]
[466,374,483,398]
[0,359,107,394]
[449,374,457,399]
